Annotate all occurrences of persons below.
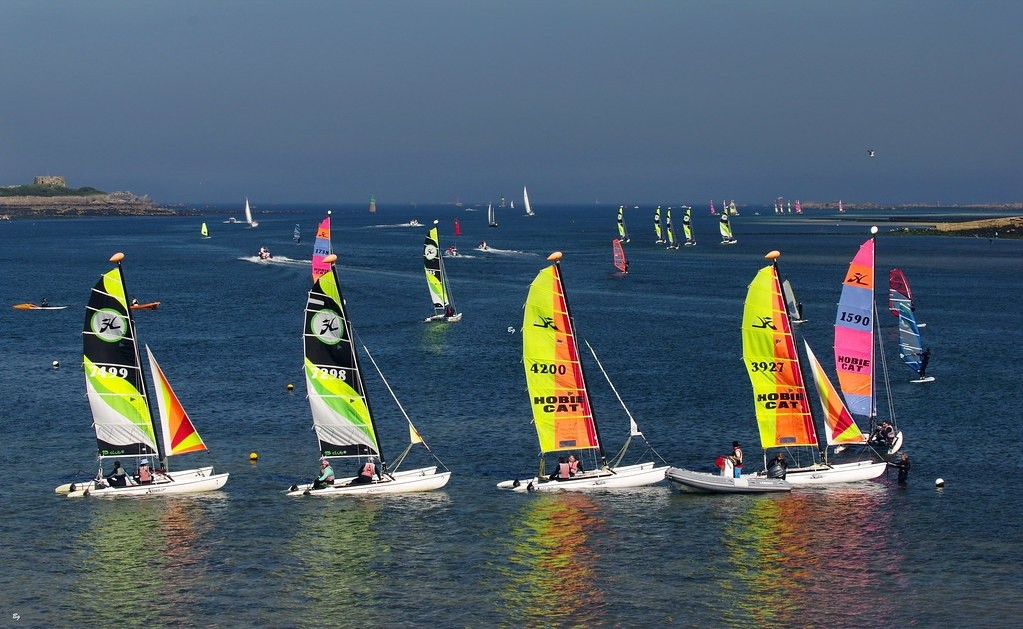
[481,240,486,249]
[103,460,126,486]
[768,452,787,470]
[881,421,894,442]
[41,298,47,306]
[449,246,456,255]
[132,297,139,306]
[259,246,273,259]
[549,457,570,480]
[911,304,915,312]
[318,459,334,485]
[798,302,803,319]
[298,238,300,243]
[352,457,383,484]
[566,453,585,477]
[732,441,744,478]
[916,348,931,379]
[446,305,455,317]
[139,459,154,484]
[898,453,910,482]
[625,260,629,271]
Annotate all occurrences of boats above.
[129,302,161,310]
[259,247,272,259]
[408,219,420,226]
[466,207,472,211]
[13,304,66,313]
[444,241,489,257]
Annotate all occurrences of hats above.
[114,461,120,465]
[568,456,575,462]
[558,456,566,462]
[140,458,149,464]
[368,457,374,462]
[732,441,739,447]
[321,461,329,465]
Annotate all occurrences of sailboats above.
[496,250,671,494]
[773,198,803,215]
[245,197,258,229]
[887,268,934,384]
[664,250,888,490]
[613,240,628,274]
[200,223,211,240]
[831,226,904,458]
[487,186,533,227]
[52,252,229,499]
[285,211,451,498]
[617,200,740,249]
[837,201,846,213]
[421,220,463,322]
